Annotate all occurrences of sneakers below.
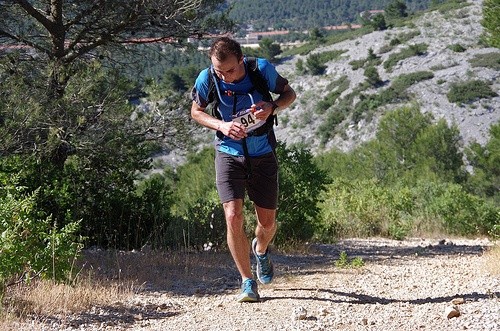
[252,238,274,284]
[238,277,259,301]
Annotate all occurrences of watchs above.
[271,100,279,115]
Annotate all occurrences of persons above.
[191,39,297,302]
